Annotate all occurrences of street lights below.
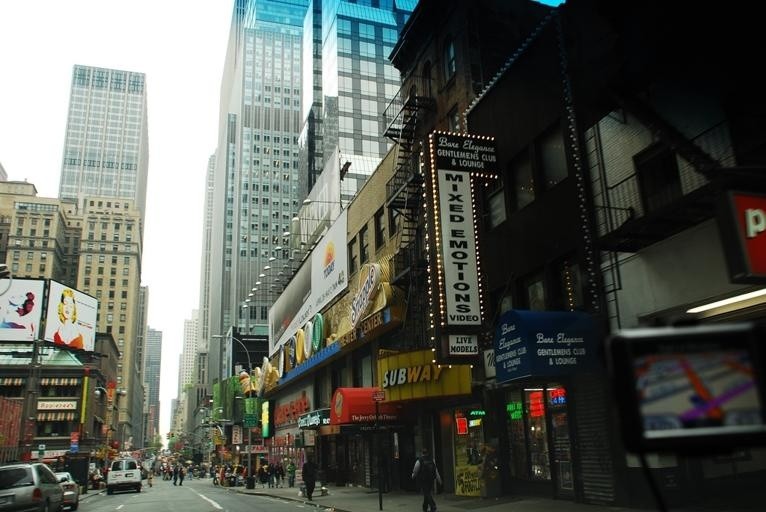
[94,386,109,457]
[211,335,255,489]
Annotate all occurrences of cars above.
[0,463,79,511]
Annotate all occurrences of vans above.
[106,459,141,494]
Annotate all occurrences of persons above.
[411,448,442,512]
[302,456,318,501]
[54,288,84,350]
[160,457,296,488]
[0,292,35,329]
[148,468,153,487]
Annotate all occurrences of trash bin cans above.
[246,477,255,489]
[79,483,84,495]
